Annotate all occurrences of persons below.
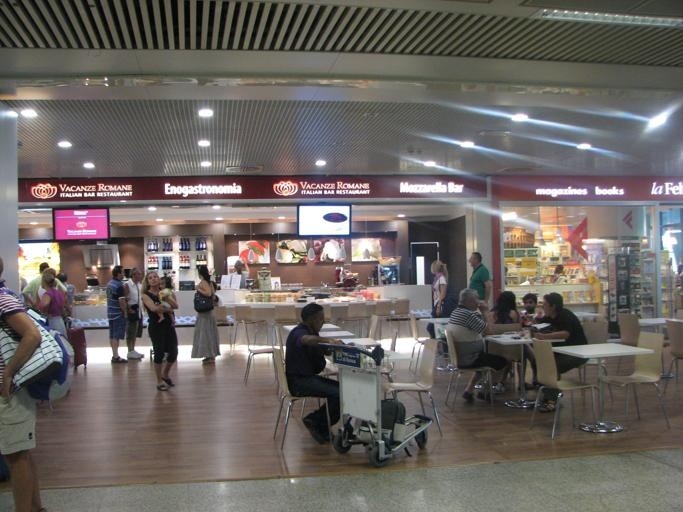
[519,264,564,286]
[0,254,76,512]
[285,303,339,446]
[468,251,491,324]
[532,293,589,413]
[449,287,508,404]
[232,259,247,290]
[519,293,544,324]
[105,265,219,392]
[427,259,449,339]
[486,290,544,391]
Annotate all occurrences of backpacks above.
[381,397,406,446]
[22,308,76,402]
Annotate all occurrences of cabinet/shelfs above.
[503,241,608,318]
[142,235,212,291]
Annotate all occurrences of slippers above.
[157,382,168,391]
[161,376,174,387]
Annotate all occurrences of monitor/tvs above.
[179,281,195,290]
[297,204,351,238]
[52,207,111,242]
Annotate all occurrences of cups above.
[563,290,592,302]
[244,293,302,303]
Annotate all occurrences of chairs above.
[403,311,586,409]
[617,312,681,387]
[528,340,596,437]
[240,314,425,412]
[380,341,446,443]
[268,346,333,449]
[600,333,667,430]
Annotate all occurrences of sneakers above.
[303,414,335,445]
[519,380,559,413]
[477,391,493,402]
[127,350,144,359]
[202,356,215,364]
[462,390,475,402]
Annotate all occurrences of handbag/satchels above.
[127,303,139,320]
[0,316,64,389]
[426,295,458,338]
[194,293,215,314]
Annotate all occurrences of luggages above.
[65,319,87,369]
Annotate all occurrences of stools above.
[210,297,412,343]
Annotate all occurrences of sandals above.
[111,356,127,363]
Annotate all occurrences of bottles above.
[146,238,207,251]
[147,253,206,269]
